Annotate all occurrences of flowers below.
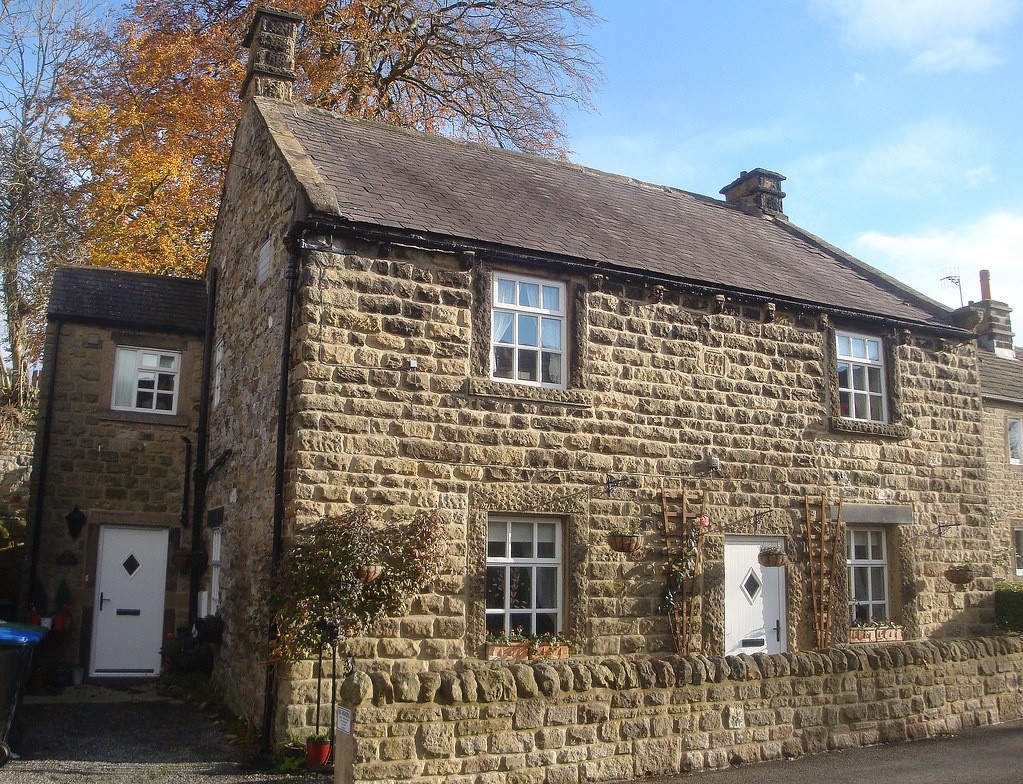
[167,632,177,641]
[486,625,529,647]
[528,631,572,662]
[852,616,876,628]
[871,616,901,632]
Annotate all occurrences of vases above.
[167,640,178,653]
[875,626,903,641]
[849,626,876,644]
[528,640,570,659]
[485,640,529,661]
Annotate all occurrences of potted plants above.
[54,607,73,631]
[172,547,193,569]
[238,720,247,737]
[184,647,199,673]
[357,556,382,584]
[197,615,224,643]
[199,644,213,675]
[269,730,302,763]
[944,562,978,584]
[306,728,332,766]
[606,518,644,551]
[758,545,788,567]
[23,606,40,625]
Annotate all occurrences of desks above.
[156,651,181,682]
